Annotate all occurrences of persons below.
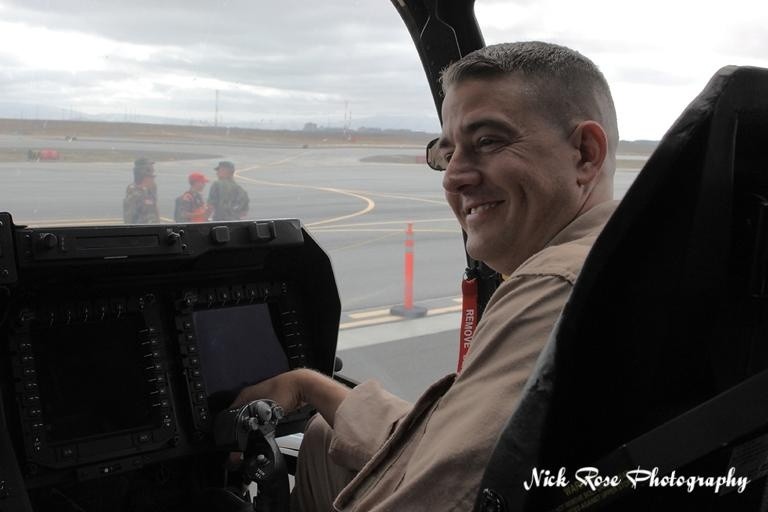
[226,41,622,512]
[123,155,161,225]
[173,173,212,223]
[206,161,249,222]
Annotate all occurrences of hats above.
[189,173,208,184]
[133,156,157,177]
[214,159,234,170]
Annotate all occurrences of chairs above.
[471,60,767,511]
